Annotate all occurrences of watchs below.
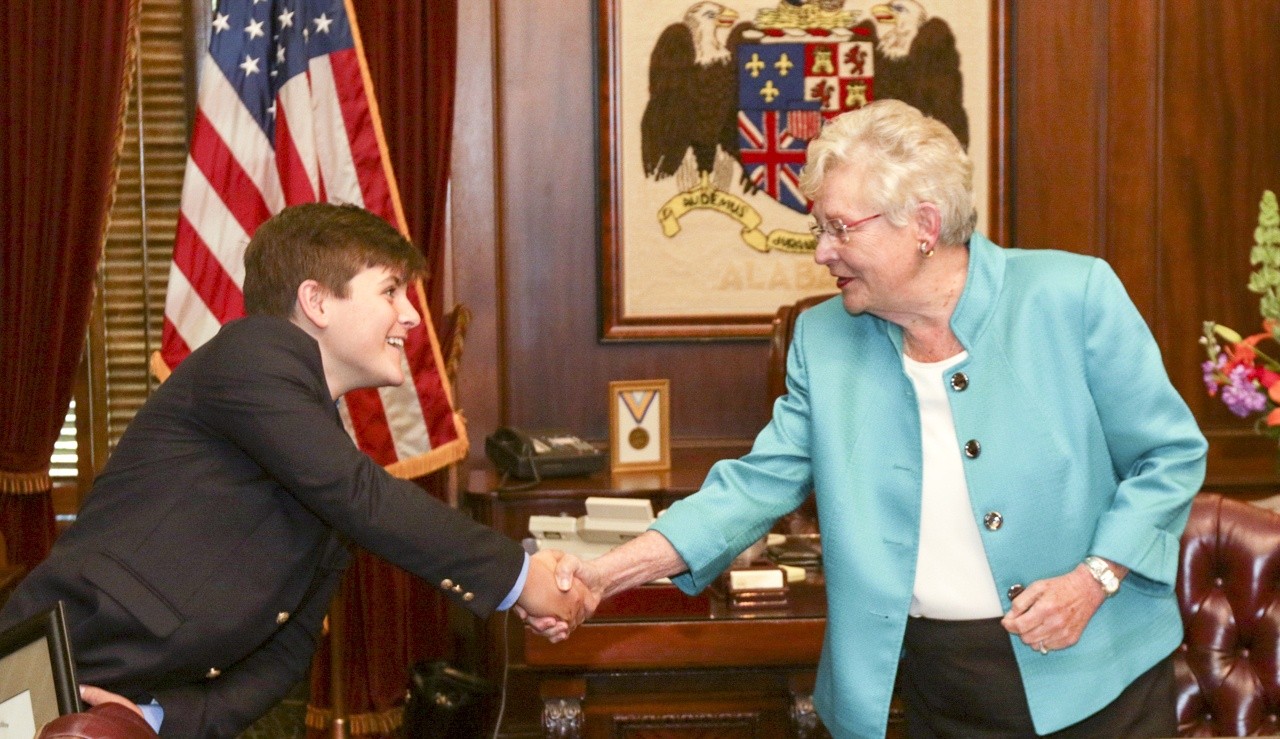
[1082,555,1120,600]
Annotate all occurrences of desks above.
[467,436,908,739]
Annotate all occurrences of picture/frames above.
[592,0,1011,346]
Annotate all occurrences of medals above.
[628,427,650,449]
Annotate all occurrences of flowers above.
[1207,195,1280,437]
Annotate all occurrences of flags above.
[159,0,469,478]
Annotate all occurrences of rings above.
[1039,641,1047,655]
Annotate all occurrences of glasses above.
[806,214,896,247]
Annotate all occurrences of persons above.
[0,202,596,739]
[514,100,1210,739]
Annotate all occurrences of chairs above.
[1168,494,1280,739]
